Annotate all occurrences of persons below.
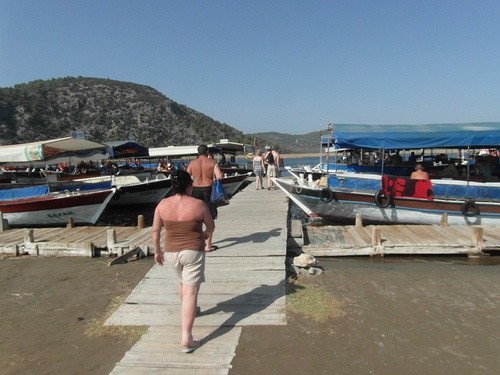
[152,169,215,352]
[25,157,190,175]
[252,137,285,190]
[410,163,429,179]
[343,148,424,166]
[187,144,224,252]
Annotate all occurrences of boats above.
[0,131,193,207]
[292,133,500,180]
[270,177,500,225]
[100,140,253,196]
[0,184,116,226]
[284,123,500,203]
[118,138,254,174]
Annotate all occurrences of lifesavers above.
[319,188,333,203]
[460,201,479,217]
[375,189,391,208]
[229,155,237,165]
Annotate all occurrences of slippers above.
[179,339,202,354]
[203,244,220,252]
